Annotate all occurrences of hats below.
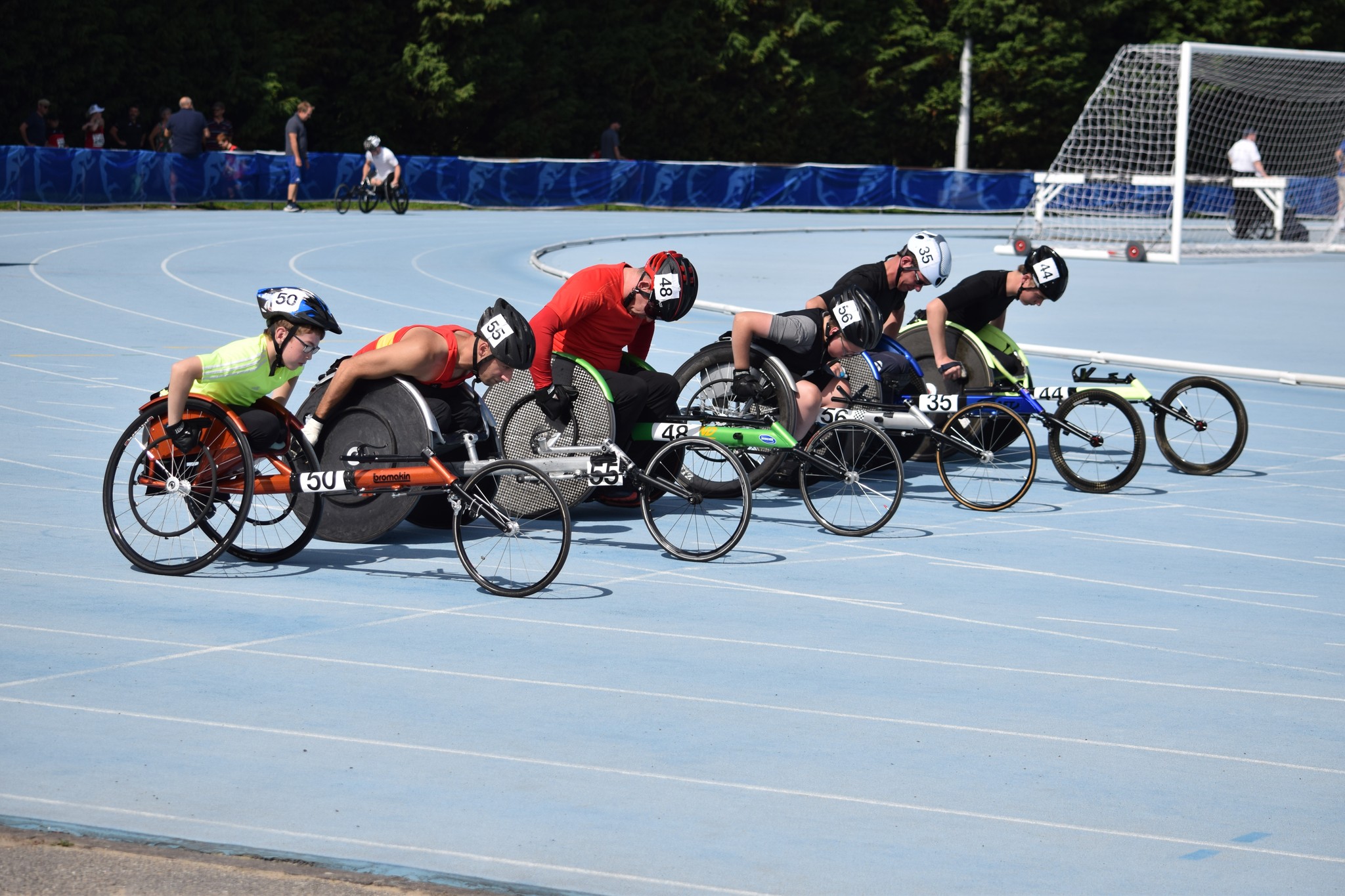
[38,100,51,105]
[88,105,105,114]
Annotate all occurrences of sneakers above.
[284,203,307,213]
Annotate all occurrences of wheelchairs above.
[333,172,409,215]
[101,394,573,600]
[893,310,1250,476]
[278,355,754,566]
[735,332,1146,497]
[668,328,1037,514]
[479,347,907,537]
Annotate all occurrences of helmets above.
[475,298,537,370]
[644,250,698,322]
[257,287,343,335]
[1024,245,1068,302]
[829,283,884,350]
[907,230,951,288]
[364,135,381,151]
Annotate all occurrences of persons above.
[358,134,401,188]
[805,231,952,409]
[596,118,626,161]
[528,250,699,458]
[731,285,884,439]
[19,97,247,209]
[282,102,315,213]
[1333,138,1345,233]
[145,285,344,478]
[1227,128,1270,239]
[926,245,1069,380]
[295,296,537,461]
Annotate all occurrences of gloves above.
[289,413,325,467]
[164,418,198,455]
[730,368,764,403]
[533,384,579,421]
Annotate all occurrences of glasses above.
[285,327,320,354]
[44,106,50,109]
[909,259,932,286]
[840,336,865,357]
[644,289,662,320]
